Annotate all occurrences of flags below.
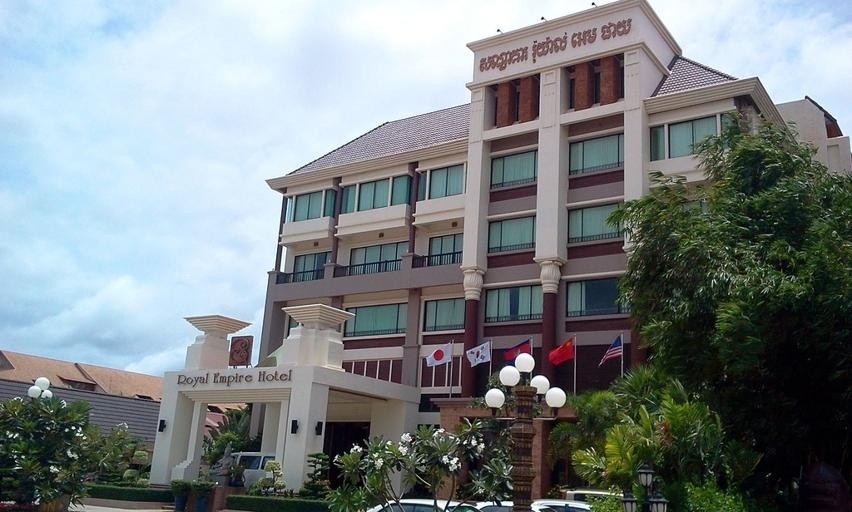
[466,341,491,368]
[596,335,622,369]
[425,343,453,367]
[503,339,532,366]
[547,336,575,366]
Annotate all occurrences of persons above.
[217,448,234,475]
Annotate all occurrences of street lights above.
[25,375,56,401]
[483,349,569,512]
[620,460,671,512]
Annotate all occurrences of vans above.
[210,449,276,494]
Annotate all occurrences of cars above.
[361,488,626,512]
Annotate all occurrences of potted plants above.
[191,480,217,512]
[0,393,107,512]
[171,479,192,512]
[228,464,246,487]
